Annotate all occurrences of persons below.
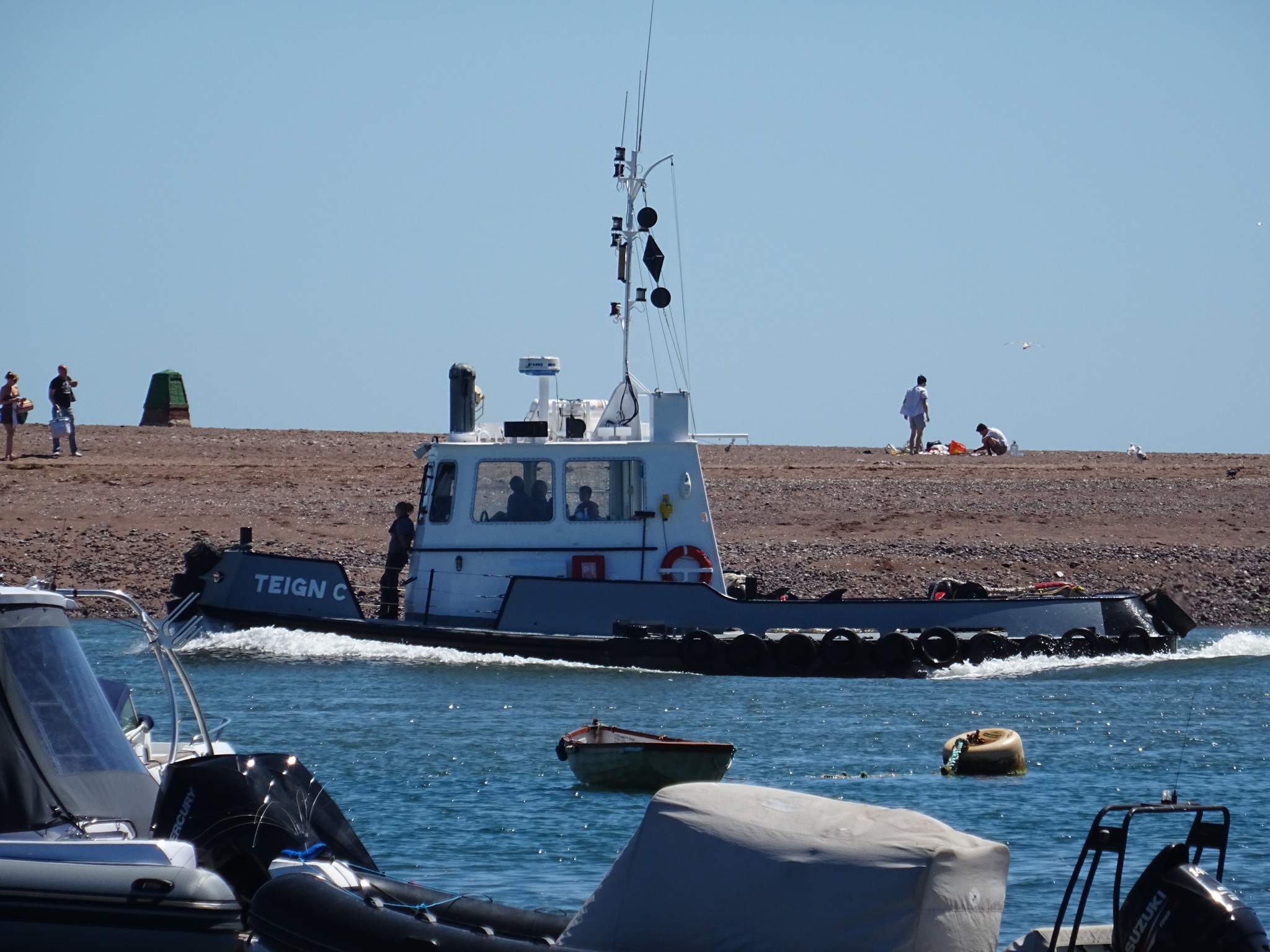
[0,372,26,459]
[378,501,415,619]
[489,476,548,522]
[48,365,82,457]
[972,423,1007,456]
[900,374,930,455]
[573,486,599,521]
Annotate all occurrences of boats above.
[246,779,1270,952]
[165,2,1198,680]
[555,719,737,794]
[0,517,381,952]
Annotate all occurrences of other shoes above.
[53,451,60,456]
[72,452,81,457]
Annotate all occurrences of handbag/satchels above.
[949,440,966,456]
[49,410,71,438]
[885,444,897,454]
[16,396,34,413]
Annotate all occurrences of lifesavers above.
[871,632,916,676]
[680,630,718,671]
[730,633,769,676]
[967,631,1006,665]
[776,633,817,678]
[818,627,864,675]
[917,626,959,669]
[660,545,713,586]
[1020,634,1058,658]
[1115,626,1151,656]
[1060,628,1101,658]
